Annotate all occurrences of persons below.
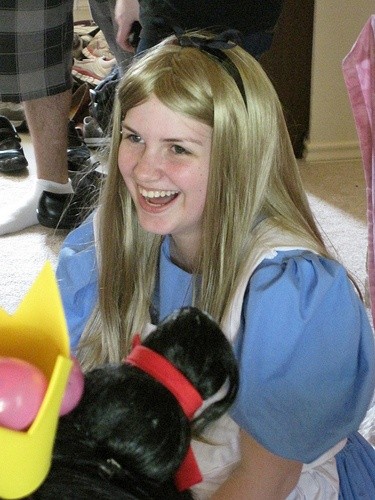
[114,0,282,159]
[0,0,137,238]
[55,28,375,500]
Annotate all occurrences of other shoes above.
[71,34,116,85]
[37,190,95,229]
[0,115,28,171]
[70,82,89,124]
[68,116,91,168]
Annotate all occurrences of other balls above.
[59,354,83,416]
[0,357,49,431]
